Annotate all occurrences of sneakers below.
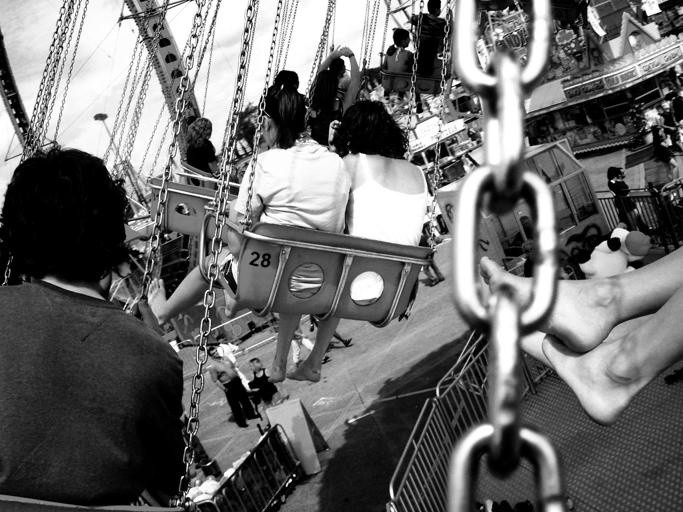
[343,338,353,348]
[238,413,261,428]
[323,354,332,364]
[429,277,445,286]
